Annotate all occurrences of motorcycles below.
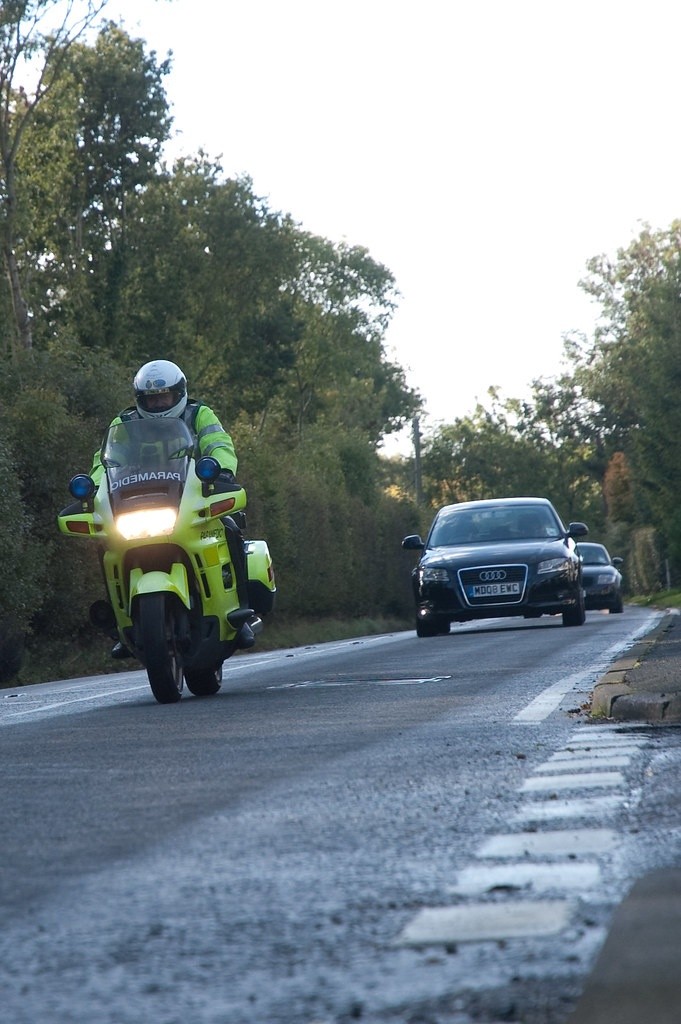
[55,415,276,703]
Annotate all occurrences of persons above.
[92,359,263,650]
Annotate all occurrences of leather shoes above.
[111,641,132,657]
[234,623,255,648]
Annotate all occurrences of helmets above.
[132,360,188,423]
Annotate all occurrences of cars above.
[524,541,625,618]
[399,496,588,637]
